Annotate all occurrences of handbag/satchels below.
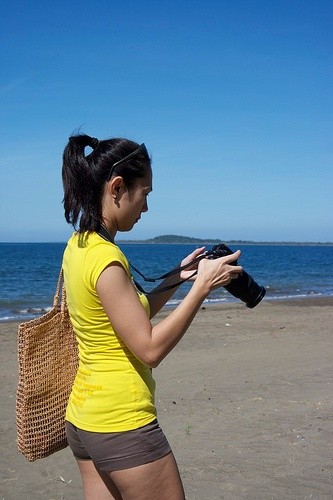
[13,264,81,462]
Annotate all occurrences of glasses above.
[107,142,150,187]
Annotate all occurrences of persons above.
[61,134,243,500]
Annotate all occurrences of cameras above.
[207,244,266,309]
